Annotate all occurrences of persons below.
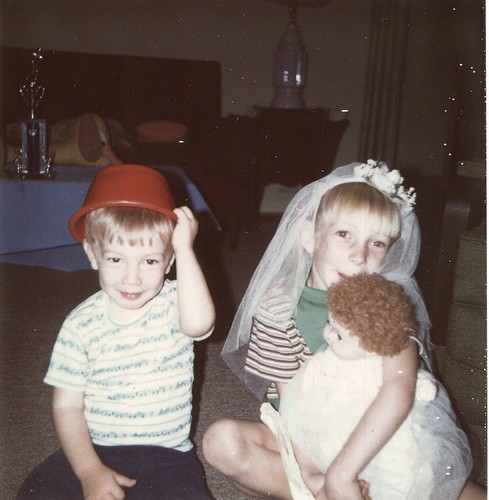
[201,160,486,499]
[15,165,219,500]
[284,273,437,500]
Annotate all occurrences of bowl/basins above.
[66,163,179,244]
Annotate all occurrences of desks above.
[0,164,215,254]
[231,114,347,247]
